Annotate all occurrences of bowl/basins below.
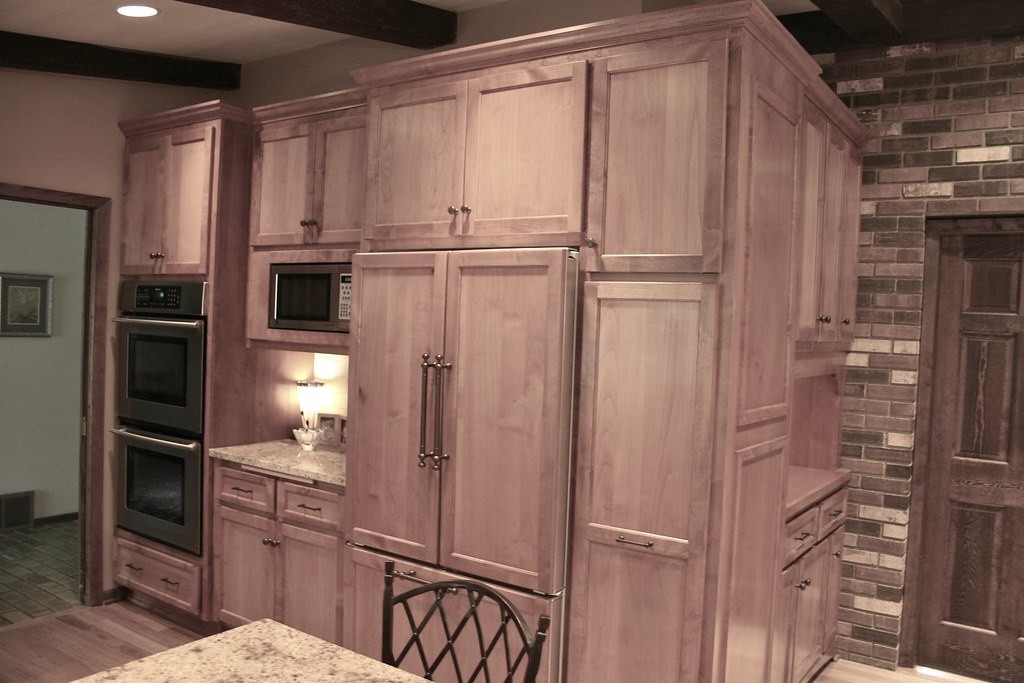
[294,428,324,451]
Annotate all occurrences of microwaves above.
[269,264,352,332]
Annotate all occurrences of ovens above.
[108,279,208,556]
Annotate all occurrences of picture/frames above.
[0,272,54,338]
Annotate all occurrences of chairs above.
[381,561,551,683]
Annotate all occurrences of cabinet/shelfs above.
[113,0,870,683]
